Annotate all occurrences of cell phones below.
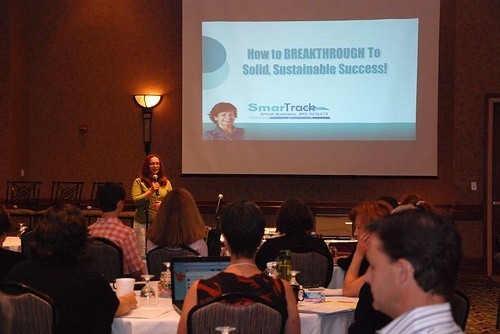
[304,291,324,300]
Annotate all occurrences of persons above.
[202,102,246,142]
[0,201,121,334]
[255,201,334,289]
[342,201,394,334]
[335,197,438,277]
[176,201,302,334]
[365,208,466,334]
[85,182,145,277]
[146,188,209,257]
[130,154,172,256]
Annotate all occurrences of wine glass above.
[16,222,26,242]
[140,275,158,304]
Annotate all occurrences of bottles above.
[303,292,325,303]
[263,263,278,279]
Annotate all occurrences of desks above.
[109,281,360,334]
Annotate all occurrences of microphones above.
[216,194,223,212]
[13,205,18,210]
[87,206,101,210]
[153,175,160,195]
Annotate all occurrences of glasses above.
[148,162,161,166]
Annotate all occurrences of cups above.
[113,278,135,296]
[291,285,305,304]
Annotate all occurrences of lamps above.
[134,94,162,154]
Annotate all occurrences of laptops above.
[168,257,231,317]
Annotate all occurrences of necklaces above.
[228,262,257,268]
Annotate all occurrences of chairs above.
[0,236,470,334]
[4,181,136,230]
